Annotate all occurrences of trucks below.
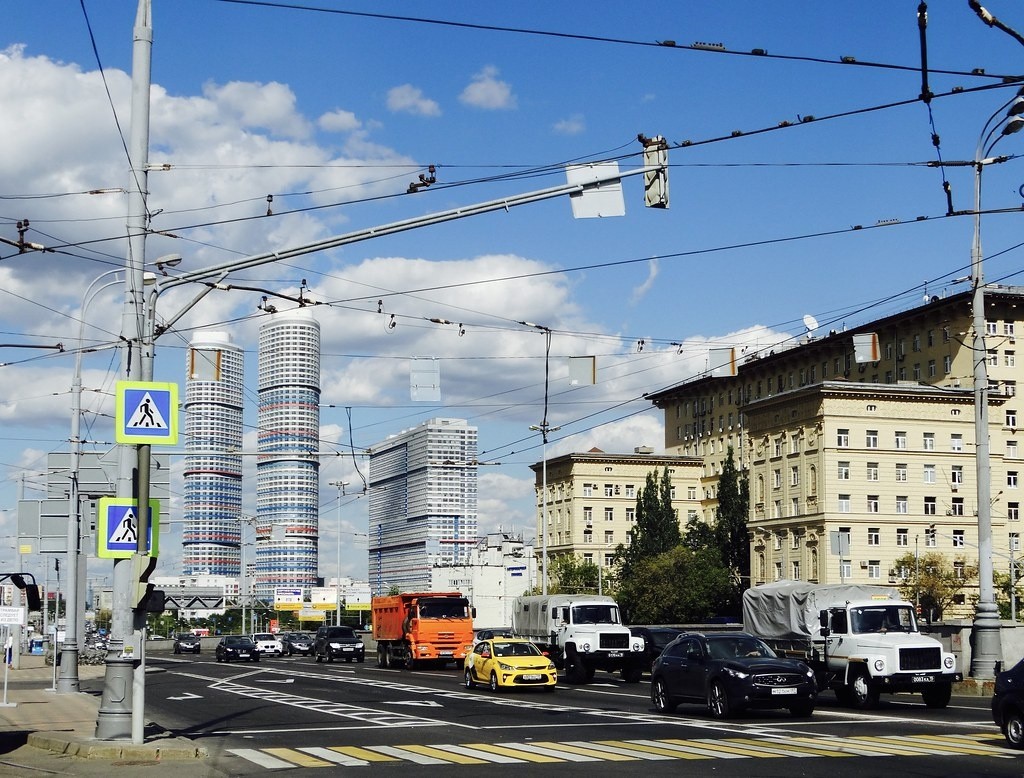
[743,578,966,710]
[510,594,646,686]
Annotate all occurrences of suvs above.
[172,633,202,653]
[314,626,367,662]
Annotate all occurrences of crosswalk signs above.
[123,389,170,439]
[109,504,150,550]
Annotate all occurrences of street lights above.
[330,481,350,626]
[528,423,562,597]
[962,98,1024,682]
[51,250,187,695]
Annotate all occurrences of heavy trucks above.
[371,590,477,670]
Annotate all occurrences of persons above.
[4,634,13,667]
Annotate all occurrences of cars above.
[650,631,820,720]
[249,633,284,658]
[473,630,516,647]
[990,659,1024,751]
[464,638,558,692]
[630,627,684,675]
[215,634,260,662]
[280,631,316,656]
